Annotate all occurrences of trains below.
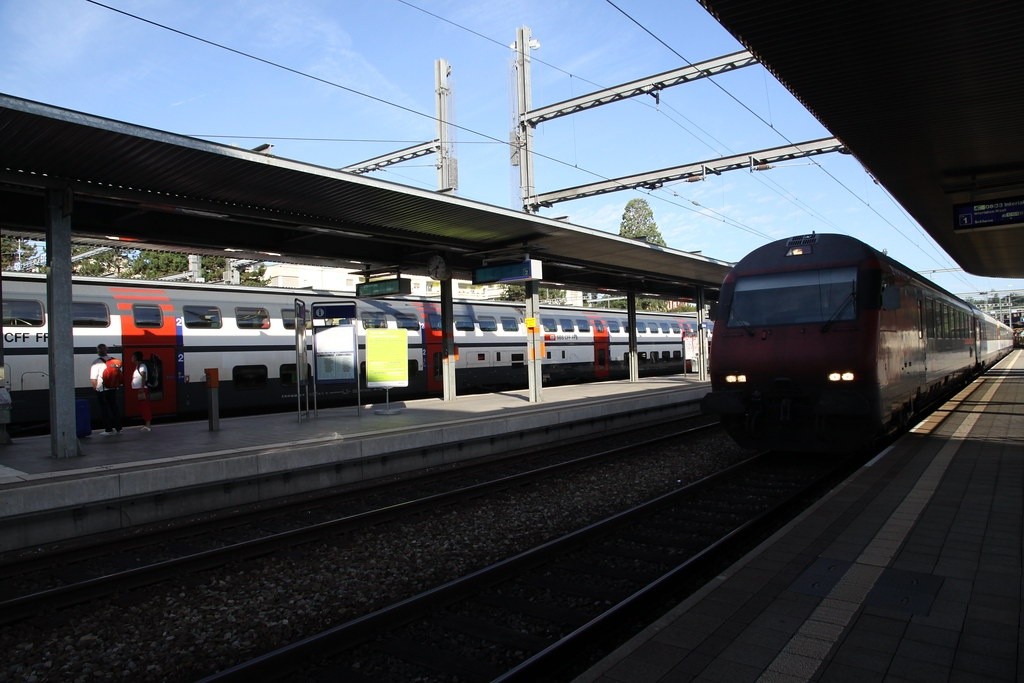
[2,273,716,442]
[709,233,1018,455]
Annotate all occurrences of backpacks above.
[97,357,124,390]
[137,360,158,387]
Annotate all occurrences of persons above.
[130,351,153,432]
[90,344,124,435]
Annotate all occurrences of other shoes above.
[116,429,125,434]
[140,426,152,432]
[99,431,113,435]
